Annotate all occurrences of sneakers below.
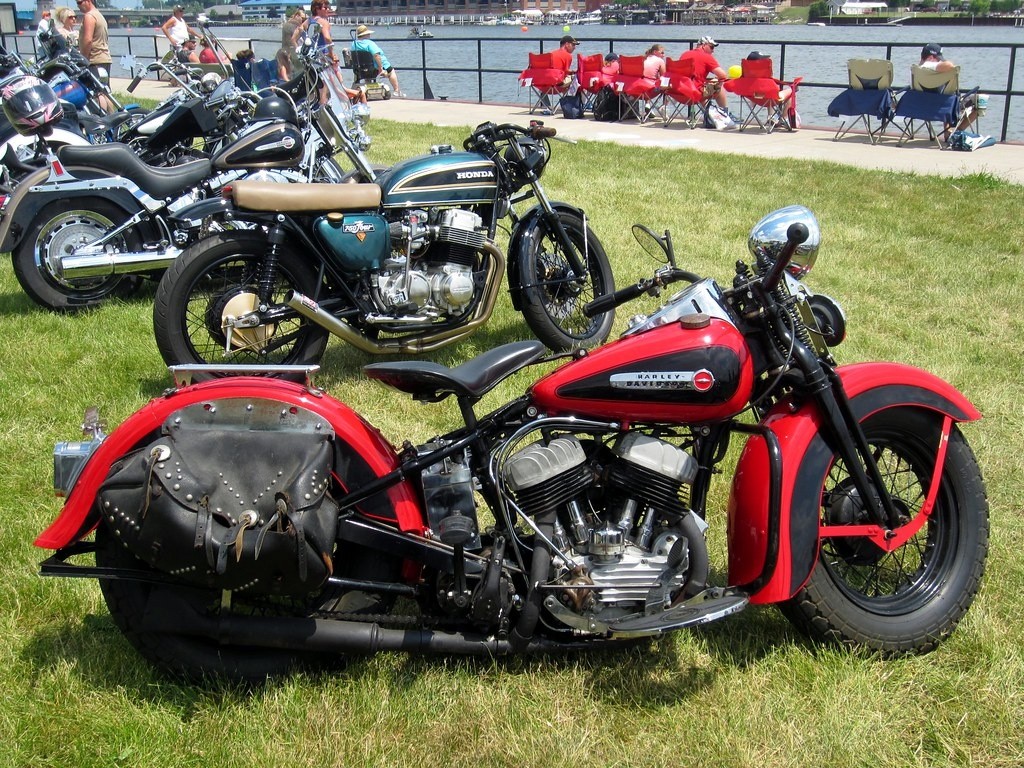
[392,90,407,99]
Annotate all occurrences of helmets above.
[3,76,64,136]
[246,93,298,124]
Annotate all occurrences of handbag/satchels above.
[949,130,995,151]
[561,93,585,120]
[704,102,735,132]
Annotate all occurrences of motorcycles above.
[0,21,387,314]
[29,207,992,692]
[154,120,618,387]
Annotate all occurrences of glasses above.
[321,6,329,10]
[78,0,84,5]
[65,16,76,20]
[707,43,715,50]
[657,50,664,54]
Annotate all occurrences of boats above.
[807,23,826,27]
[478,16,534,26]
[406,25,434,39]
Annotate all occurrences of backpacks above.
[592,84,626,124]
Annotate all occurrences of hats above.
[174,4,184,12]
[746,50,771,60]
[697,36,720,47]
[285,6,298,18]
[922,42,945,63]
[356,25,375,36]
[181,36,196,45]
[605,53,618,61]
[559,35,580,46]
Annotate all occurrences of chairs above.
[827,59,979,150]
[518,52,730,129]
[723,59,803,134]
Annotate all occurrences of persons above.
[747,50,794,128]
[918,42,979,141]
[600,52,620,87]
[274,0,367,107]
[35,12,51,57]
[177,36,201,63]
[642,43,669,121]
[54,6,79,49]
[161,4,202,87]
[349,25,406,98]
[75,0,114,114]
[678,36,744,127]
[548,35,592,112]
[237,49,255,63]
[199,34,232,63]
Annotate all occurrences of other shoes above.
[777,119,789,126]
[767,115,776,127]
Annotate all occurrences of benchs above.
[184,63,234,78]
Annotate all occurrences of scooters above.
[350,29,392,102]
